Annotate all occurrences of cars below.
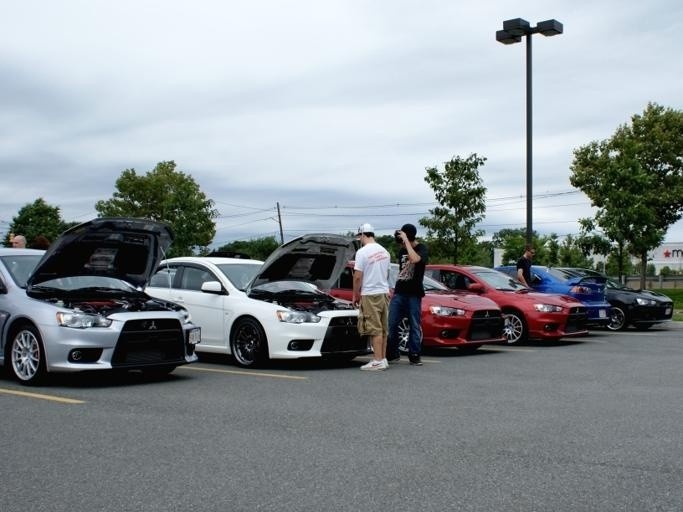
[138,231,375,365]
[1,215,202,386]
[299,261,508,356]
[425,264,591,346]
[493,265,612,330]
[554,268,675,332]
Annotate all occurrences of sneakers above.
[385,354,400,364]
[407,355,423,366]
[359,356,390,371]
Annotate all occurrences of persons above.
[9,234,26,250]
[28,235,50,249]
[515,244,537,293]
[384,223,427,367]
[351,221,392,372]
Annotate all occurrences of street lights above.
[495,16,563,262]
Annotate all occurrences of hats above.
[354,223,375,236]
[397,223,418,237]
